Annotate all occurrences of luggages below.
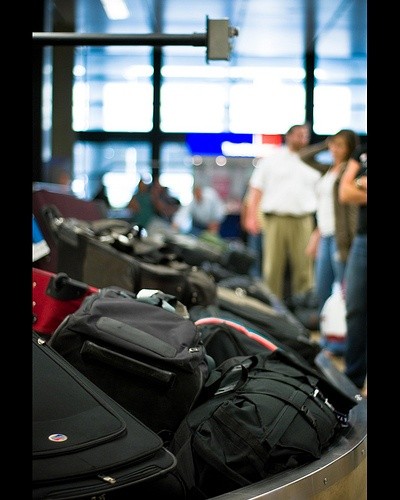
[31,267,97,337]
[30,330,177,498]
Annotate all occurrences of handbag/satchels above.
[49,286,215,432]
[167,350,358,499]
[189,305,309,369]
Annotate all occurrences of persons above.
[240,125,322,302]
[92,169,245,240]
[304,130,359,315]
[338,140,368,391]
